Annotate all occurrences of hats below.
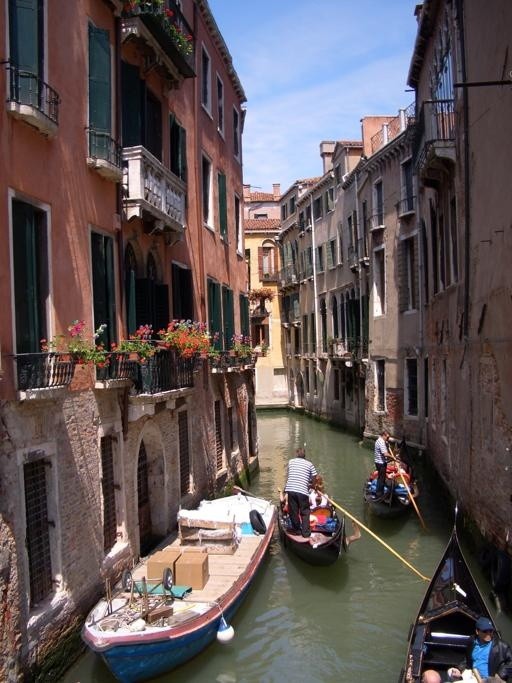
[476,616,496,633]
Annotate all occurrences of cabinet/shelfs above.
[179,515,238,555]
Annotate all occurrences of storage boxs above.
[147,545,209,590]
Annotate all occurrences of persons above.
[284,447,318,539]
[277,486,290,514]
[307,473,324,496]
[373,429,398,499]
[443,615,511,683]
[421,669,441,683]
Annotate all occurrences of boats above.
[398,500,512,683]
[362,437,420,519]
